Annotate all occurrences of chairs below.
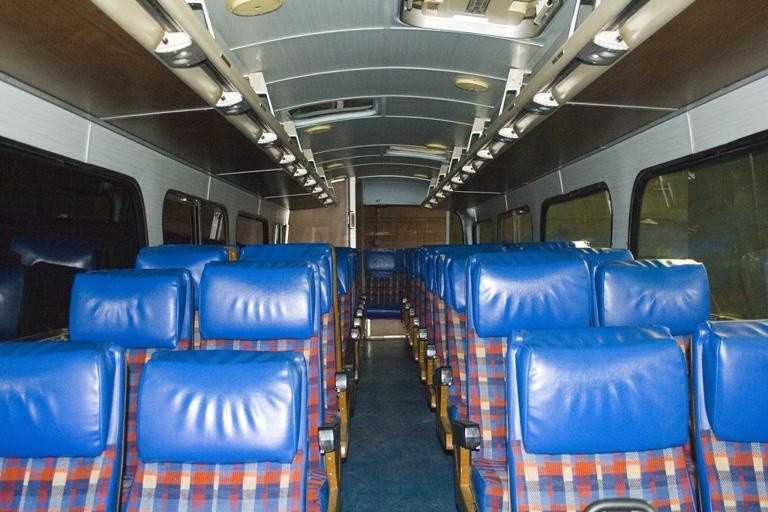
[404,242,768,511]
[362,248,405,318]
[0,239,361,512]
